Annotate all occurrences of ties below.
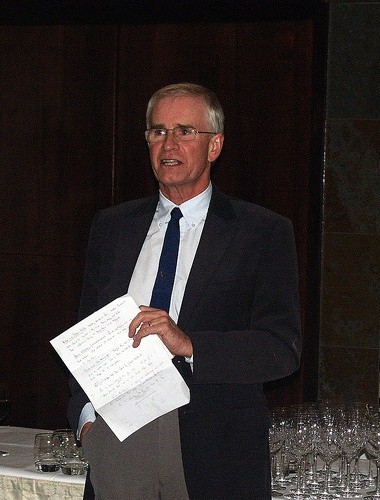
[149,207,183,313]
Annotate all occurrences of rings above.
[148,321,152,326]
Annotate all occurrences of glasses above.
[144,126,218,143]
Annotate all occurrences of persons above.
[66,82,303,500]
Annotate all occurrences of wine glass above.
[269,400,380,500]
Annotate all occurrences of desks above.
[0,424,380,500]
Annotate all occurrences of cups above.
[52,429,74,464]
[34,433,60,472]
[59,434,88,476]
